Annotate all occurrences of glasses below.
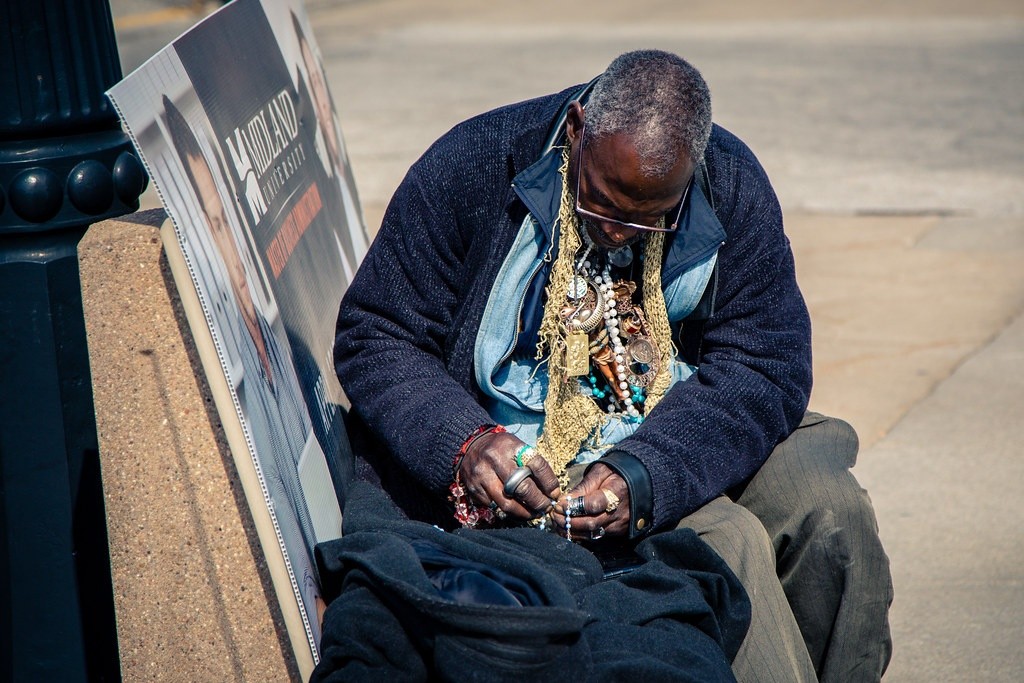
[575,123,693,233]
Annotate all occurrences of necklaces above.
[538,492,572,541]
[524,149,661,424]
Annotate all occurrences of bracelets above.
[448,424,509,529]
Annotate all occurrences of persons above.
[333,50,895,683]
[161,9,371,657]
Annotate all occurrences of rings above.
[488,501,497,511]
[587,528,605,540]
[570,496,587,517]
[601,487,620,513]
[503,444,542,498]
